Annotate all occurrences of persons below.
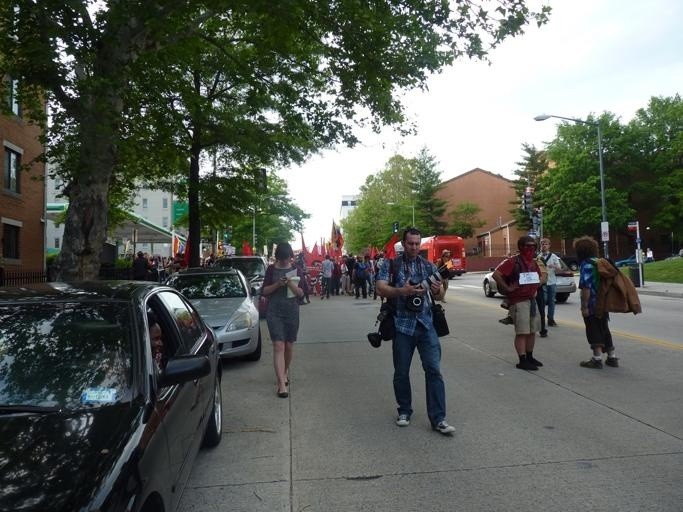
[533,240,549,337]
[646,247,653,263]
[538,237,561,326]
[366,229,456,434]
[575,235,618,369]
[261,242,308,398]
[492,235,543,371]
[117,311,163,388]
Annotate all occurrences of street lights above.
[387,202,415,228]
[533,113,611,261]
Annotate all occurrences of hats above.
[441,249,451,257]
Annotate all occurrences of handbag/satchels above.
[432,304,449,337]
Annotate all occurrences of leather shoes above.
[277,388,288,397]
[285,376,288,385]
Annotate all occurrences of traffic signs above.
[628,221,638,227]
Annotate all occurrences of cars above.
[613,254,651,268]
[1,279,226,510]
[663,254,680,261]
[162,267,262,360]
[483,251,578,302]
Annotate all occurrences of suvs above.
[211,254,270,319]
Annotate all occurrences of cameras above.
[411,264,452,296]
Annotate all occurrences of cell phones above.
[281,276,287,282]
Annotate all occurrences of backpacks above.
[534,254,548,286]
[588,257,603,293]
[358,263,368,278]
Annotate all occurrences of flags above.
[369,233,398,259]
[241,240,252,255]
[174,235,185,255]
[180,239,190,267]
[302,220,344,257]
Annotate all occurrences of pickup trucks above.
[553,253,577,270]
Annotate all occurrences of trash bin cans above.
[627,263,644,287]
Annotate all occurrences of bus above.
[394,236,467,279]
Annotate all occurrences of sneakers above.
[396,415,409,426]
[605,358,618,367]
[436,421,456,433]
[516,362,537,370]
[547,320,556,326]
[499,317,513,324]
[581,359,601,369]
[526,358,543,366]
[539,329,547,337]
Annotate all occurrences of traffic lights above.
[522,193,531,209]
[535,209,541,225]
